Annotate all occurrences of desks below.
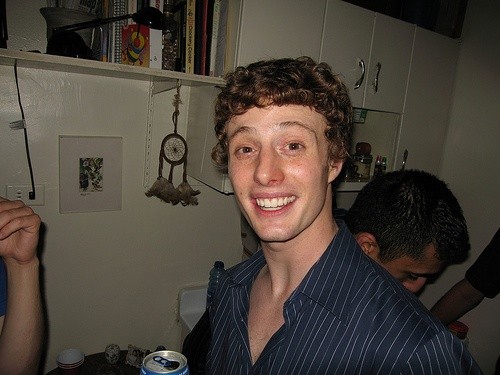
[45,349,147,375]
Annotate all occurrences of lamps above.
[46,6,172,61]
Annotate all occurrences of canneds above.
[139,350,191,375]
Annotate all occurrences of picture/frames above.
[58,135,122,214]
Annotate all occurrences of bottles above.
[379,156,387,176]
[353,141,373,180]
[162,0,180,71]
[373,155,382,180]
[207,260,226,307]
[105,344,120,364]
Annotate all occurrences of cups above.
[56,348,85,375]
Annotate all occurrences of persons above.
[180,56,483,375]
[341,168,500,375]
[0,198,48,375]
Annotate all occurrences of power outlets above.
[6,184,45,206]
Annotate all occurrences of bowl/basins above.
[40,6,97,49]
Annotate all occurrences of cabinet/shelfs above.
[0,0,470,197]
